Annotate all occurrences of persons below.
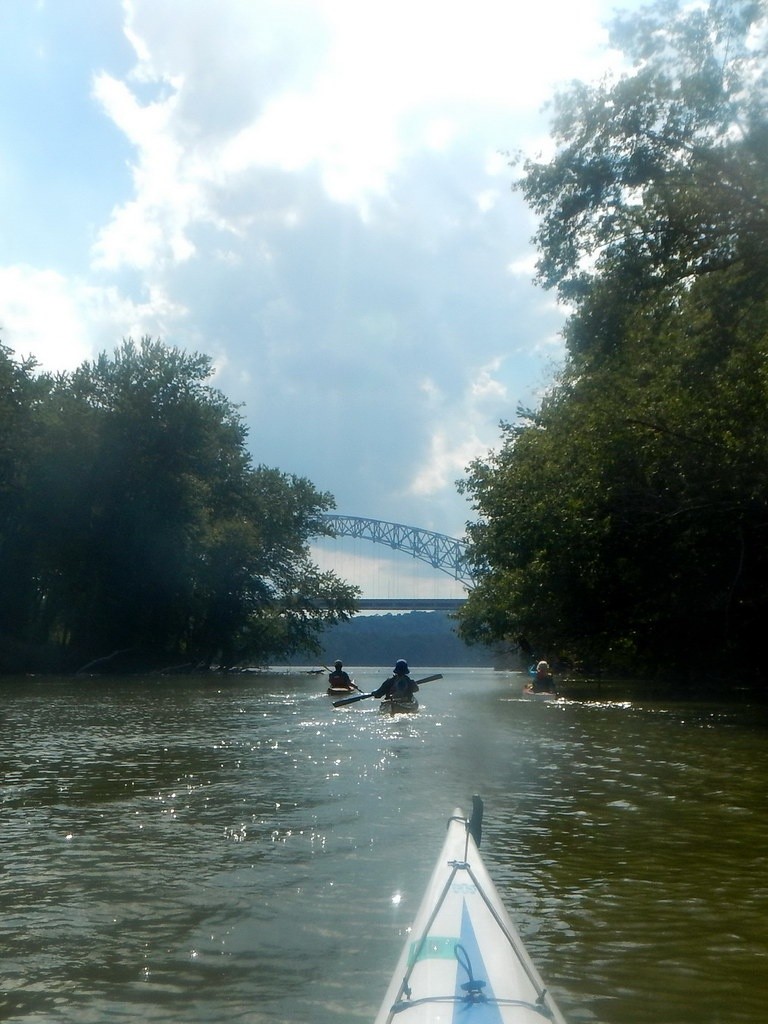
[329,660,351,690]
[371,659,419,702]
[530,661,559,693]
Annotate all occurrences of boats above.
[327,680,356,694]
[380,695,420,714]
[523,684,559,701]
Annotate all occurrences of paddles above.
[517,634,559,693]
[333,674,443,707]
[322,664,363,693]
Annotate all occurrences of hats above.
[335,660,342,668]
[393,659,410,674]
[537,661,549,672]
